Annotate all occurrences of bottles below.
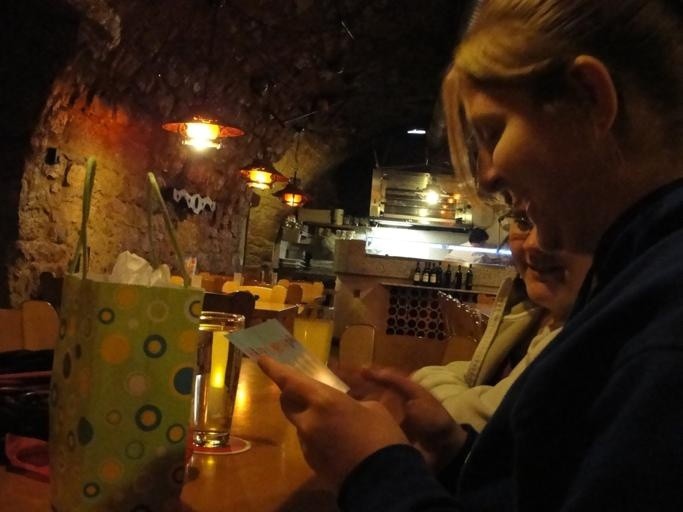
[411,260,474,291]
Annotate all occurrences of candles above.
[210,331,227,385]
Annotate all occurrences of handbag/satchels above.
[46,155,206,512]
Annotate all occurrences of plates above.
[310,258,337,269]
[279,258,307,269]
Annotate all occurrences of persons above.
[259,1,683,512]
[408,193,593,429]
[449,227,494,263]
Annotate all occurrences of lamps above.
[239,139,291,186]
[271,170,308,209]
[160,73,245,160]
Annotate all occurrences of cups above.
[185,310,247,449]
[334,208,345,225]
[183,256,198,279]
[292,304,335,369]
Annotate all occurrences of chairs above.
[171,270,325,327]
[0,309,23,352]
[21,300,60,351]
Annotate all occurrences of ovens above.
[366,167,473,227]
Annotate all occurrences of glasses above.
[496,205,531,240]
